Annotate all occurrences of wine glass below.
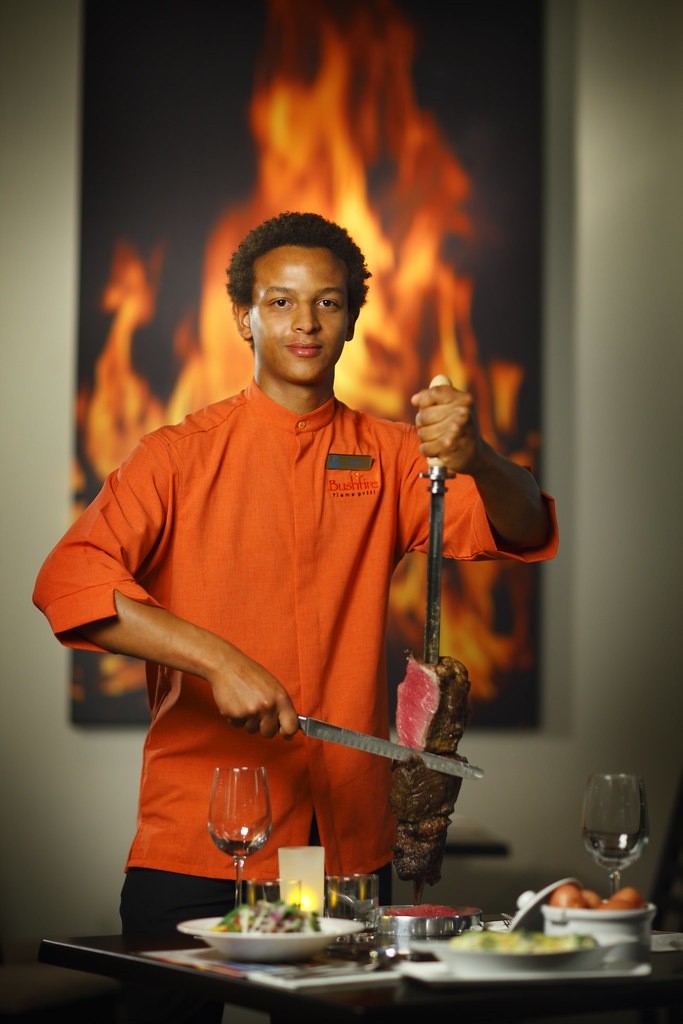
[582,772,651,898]
[207,767,271,909]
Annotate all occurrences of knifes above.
[297,714,487,783]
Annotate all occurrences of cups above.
[541,899,655,963]
[326,874,378,930]
[248,878,301,910]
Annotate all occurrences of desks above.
[40,913,683,1024]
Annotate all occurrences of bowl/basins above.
[370,905,485,953]
[177,916,364,963]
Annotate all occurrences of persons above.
[29,209,561,940]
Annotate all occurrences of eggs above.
[612,888,646,909]
[582,890,601,909]
[599,900,634,910]
[551,885,585,908]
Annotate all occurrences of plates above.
[391,960,653,983]
[409,935,639,969]
